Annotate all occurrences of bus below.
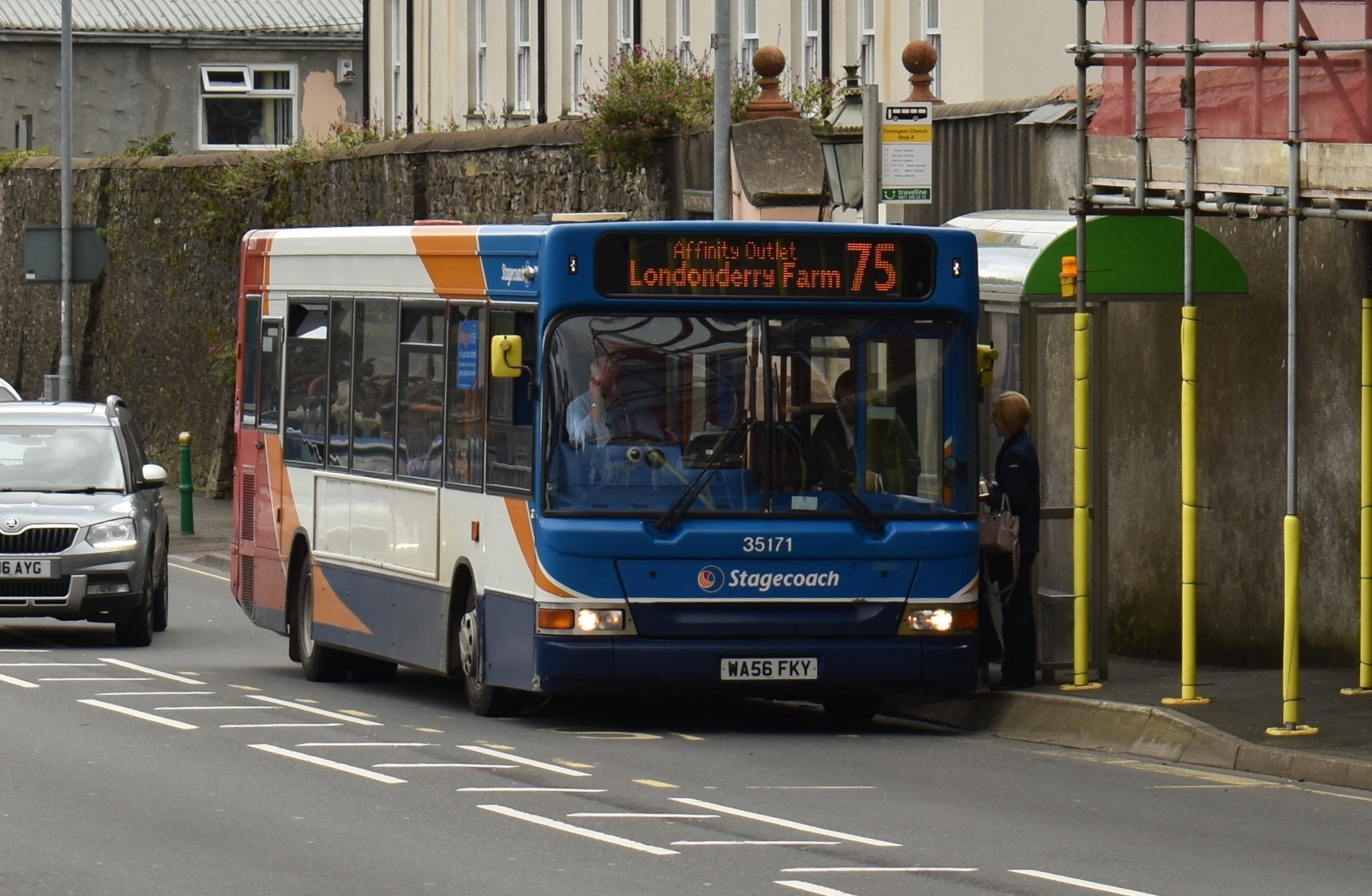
[230,208,997,721]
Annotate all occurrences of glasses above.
[834,394,857,405]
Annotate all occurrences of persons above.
[565,354,667,448]
[811,372,923,497]
[976,392,1041,690]
[289,330,455,484]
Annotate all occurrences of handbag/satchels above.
[980,492,1018,553]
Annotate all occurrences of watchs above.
[591,402,603,411]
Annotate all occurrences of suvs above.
[1,395,173,644]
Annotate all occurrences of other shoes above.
[978,653,1000,666]
[989,680,1036,691]
